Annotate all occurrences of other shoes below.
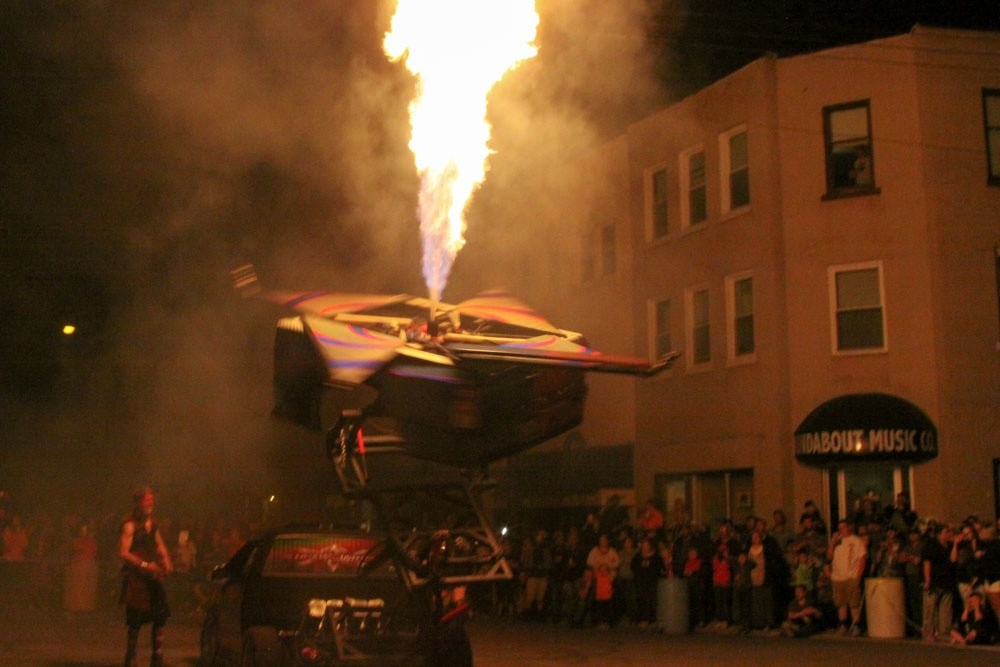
[924,636,937,642]
[836,625,847,635]
[851,625,862,637]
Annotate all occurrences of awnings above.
[795,392,939,465]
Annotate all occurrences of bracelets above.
[141,561,147,569]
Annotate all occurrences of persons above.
[0,506,262,627]
[121,490,171,667]
[470,488,1000,647]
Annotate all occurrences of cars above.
[200,528,473,667]
